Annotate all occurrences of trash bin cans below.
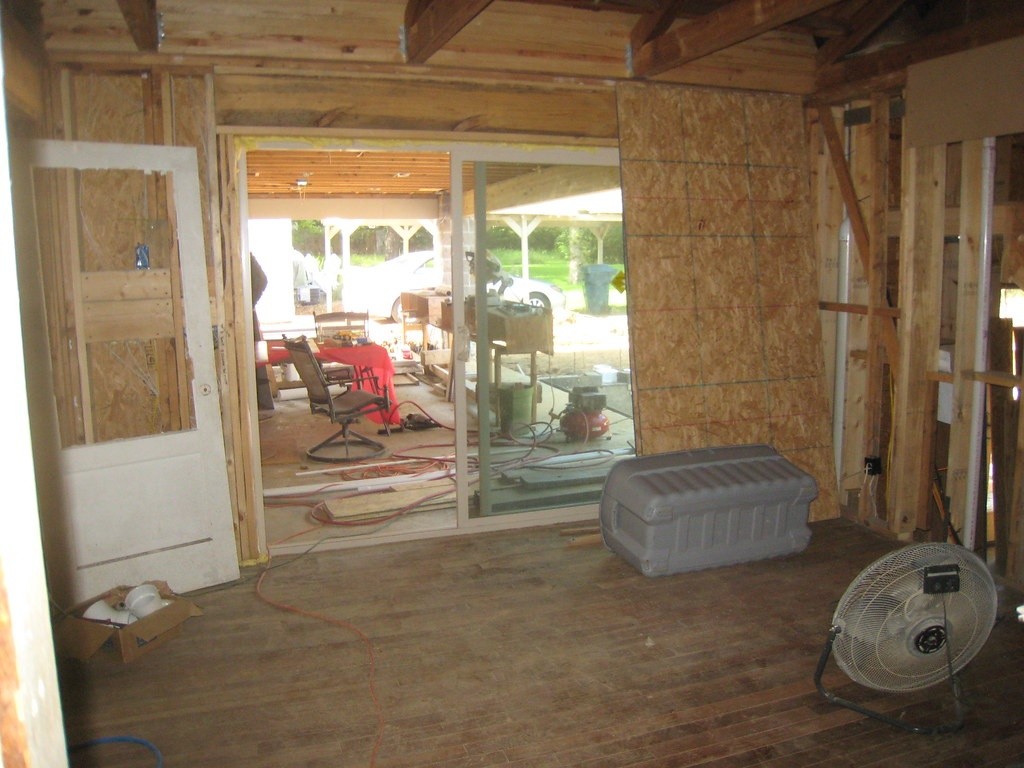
[580,263,615,313]
[498,383,535,436]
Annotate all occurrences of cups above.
[316,326,324,344]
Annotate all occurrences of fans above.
[814,543,998,735]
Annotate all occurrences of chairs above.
[282,334,353,392]
[283,339,395,462]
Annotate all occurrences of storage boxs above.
[55,581,202,662]
[599,442,817,579]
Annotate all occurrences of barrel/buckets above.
[497,384,534,436]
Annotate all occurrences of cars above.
[343,250,570,329]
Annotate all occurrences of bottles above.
[402,343,410,360]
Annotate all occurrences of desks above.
[441,297,552,385]
[402,287,452,350]
